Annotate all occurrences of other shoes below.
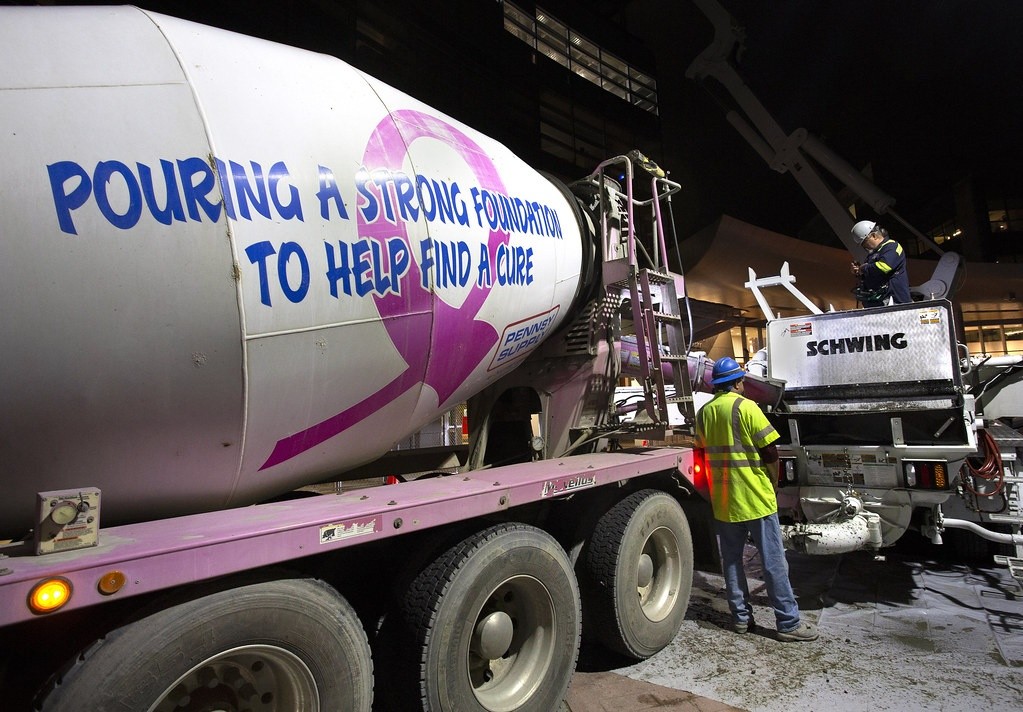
[733,618,756,634]
[775,623,819,642]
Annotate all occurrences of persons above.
[850,220,912,308]
[694,357,819,643]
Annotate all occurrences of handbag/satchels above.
[853,283,890,308]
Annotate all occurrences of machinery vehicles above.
[622,253,1023,605]
[2,2,695,712]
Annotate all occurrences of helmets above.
[709,356,746,384]
[850,220,876,247]
